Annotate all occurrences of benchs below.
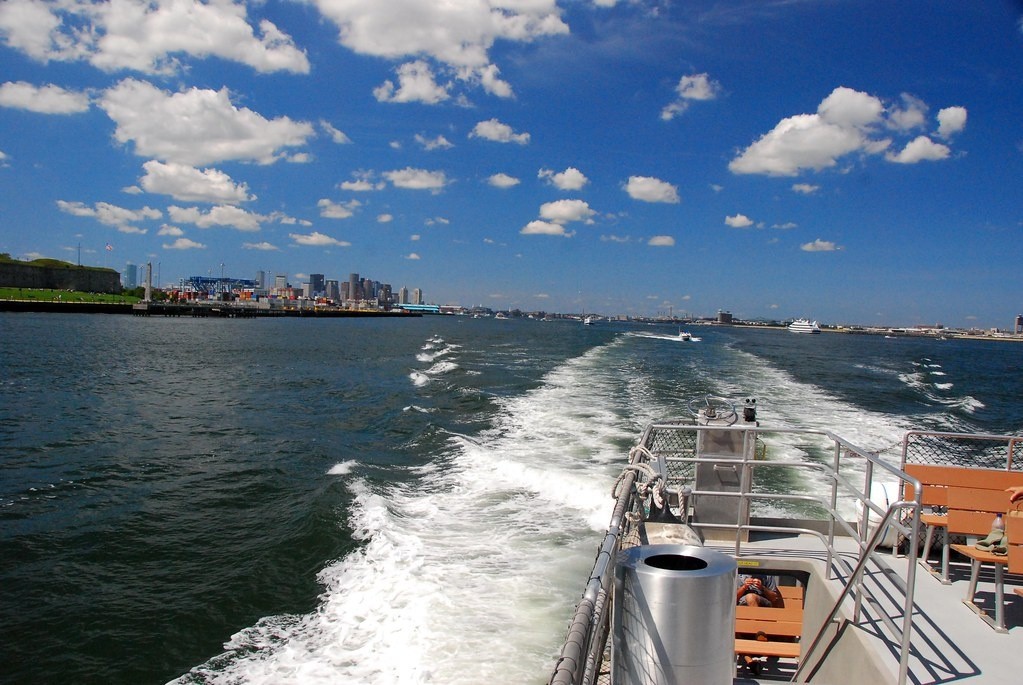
[733,586,805,656]
[904,464,1023,633]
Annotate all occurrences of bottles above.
[992,513,1005,535]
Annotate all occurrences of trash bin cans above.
[610,541,738,685]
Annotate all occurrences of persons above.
[1004,485,1023,504]
[736,568,785,678]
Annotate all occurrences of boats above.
[678,332,693,341]
[786,319,822,334]
[583,316,597,326]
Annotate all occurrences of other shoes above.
[749,660,763,674]
[768,662,780,674]
